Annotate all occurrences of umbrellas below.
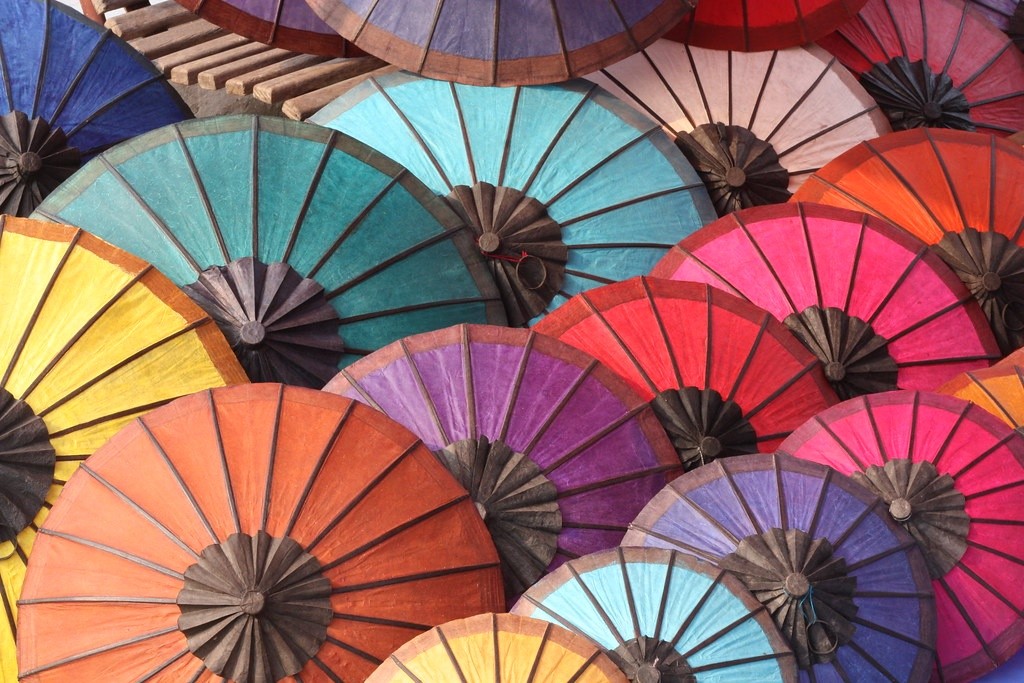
[0,0,1024,683]
